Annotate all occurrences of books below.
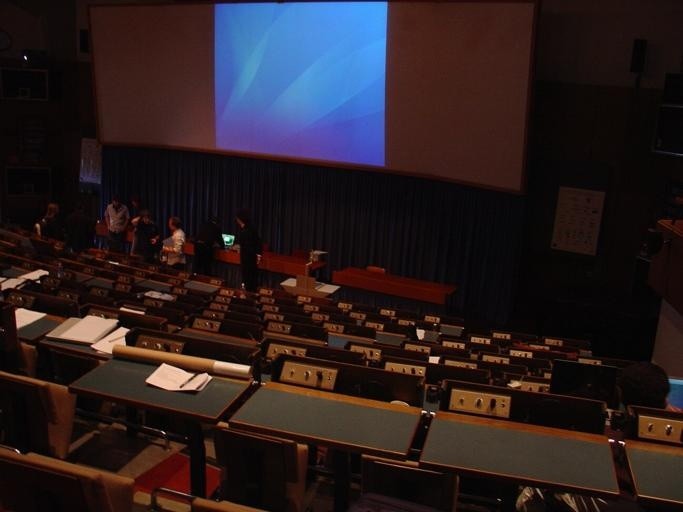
[143,361,210,393]
[44,314,83,343]
[60,314,120,345]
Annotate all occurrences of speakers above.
[628,39,647,73]
[0,66,49,101]
[662,72,683,104]
[4,166,51,199]
[648,104,683,157]
[80,31,88,54]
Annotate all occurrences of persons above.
[191,214,227,277]
[60,198,98,253]
[160,216,187,269]
[34,201,64,240]
[102,195,130,254]
[615,359,683,414]
[130,210,162,266]
[235,214,258,291]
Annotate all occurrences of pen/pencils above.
[108,335,125,343]
[179,371,199,388]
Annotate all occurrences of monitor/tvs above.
[222,233,235,247]
[665,378,683,410]
[549,359,618,409]
